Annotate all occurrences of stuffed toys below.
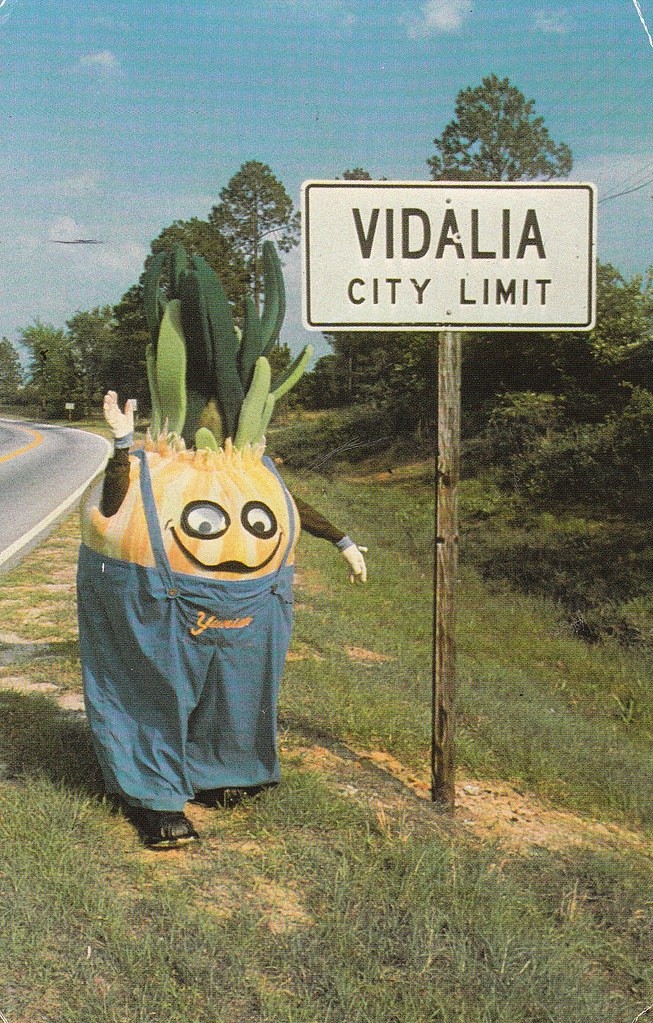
[77,241,367,847]
